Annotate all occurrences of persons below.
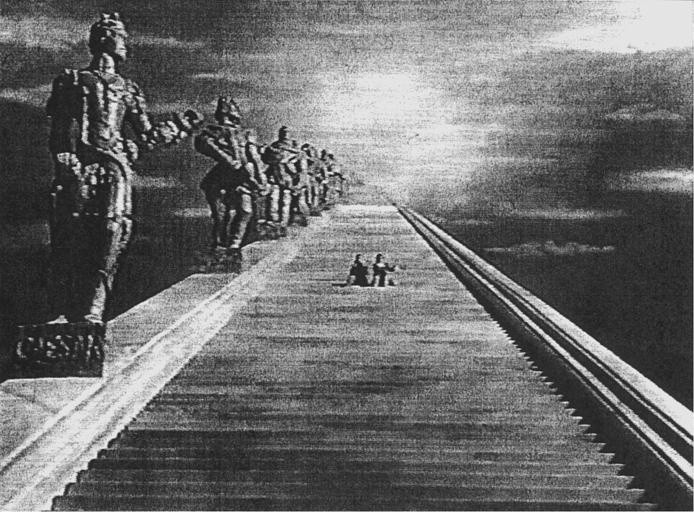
[263,125,365,232]
[45,11,205,325]
[372,254,399,288]
[347,253,369,285]
[195,95,270,252]
